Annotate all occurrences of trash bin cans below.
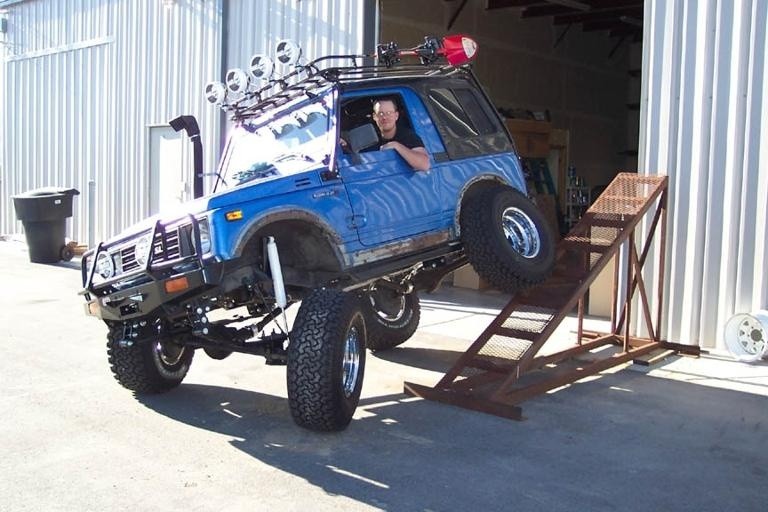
[12,187,80,264]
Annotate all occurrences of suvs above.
[78,31,559,435]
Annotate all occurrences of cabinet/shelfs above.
[496,107,591,230]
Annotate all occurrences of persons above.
[339,95,429,171]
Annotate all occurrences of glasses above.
[374,110,391,117]
